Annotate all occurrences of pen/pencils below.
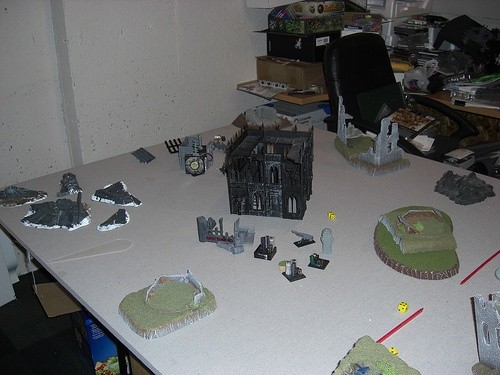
[460,250,500,285]
[376,308,424,343]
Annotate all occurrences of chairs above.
[322,32,479,159]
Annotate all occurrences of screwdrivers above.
[288,91,315,95]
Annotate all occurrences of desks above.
[237,57,500,119]
[0,121,500,375]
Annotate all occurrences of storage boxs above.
[256,29,339,63]
[256,101,331,120]
[256,55,325,90]
[32,282,130,375]
[343,12,388,33]
